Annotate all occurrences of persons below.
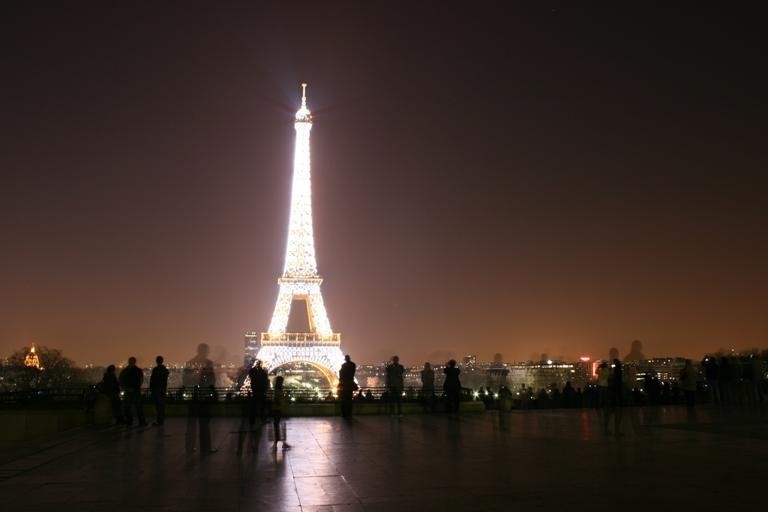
[249,360,270,424]
[443,359,461,412]
[478,353,768,439]
[339,354,356,420]
[100,356,169,427]
[272,376,290,451]
[385,355,406,418]
[421,362,435,414]
